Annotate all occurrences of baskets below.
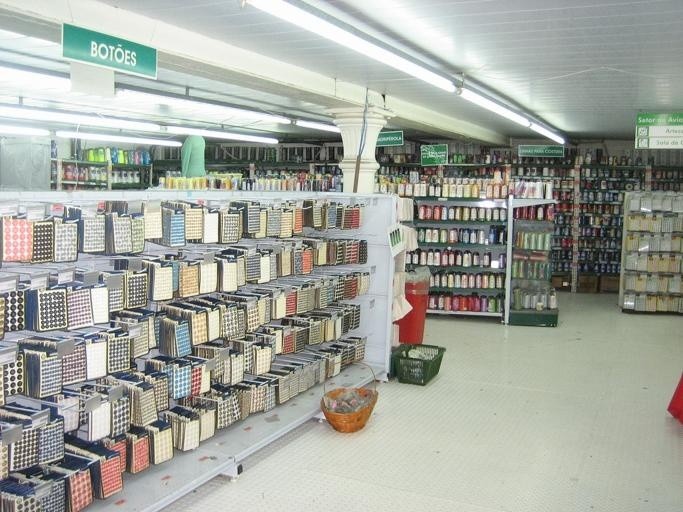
[391,344,446,385]
[321,361,378,432]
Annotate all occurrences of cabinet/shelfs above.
[1,189,399,512]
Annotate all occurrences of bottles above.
[242,173,312,192]
[312,143,611,313]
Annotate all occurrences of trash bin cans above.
[393,275,430,344]
